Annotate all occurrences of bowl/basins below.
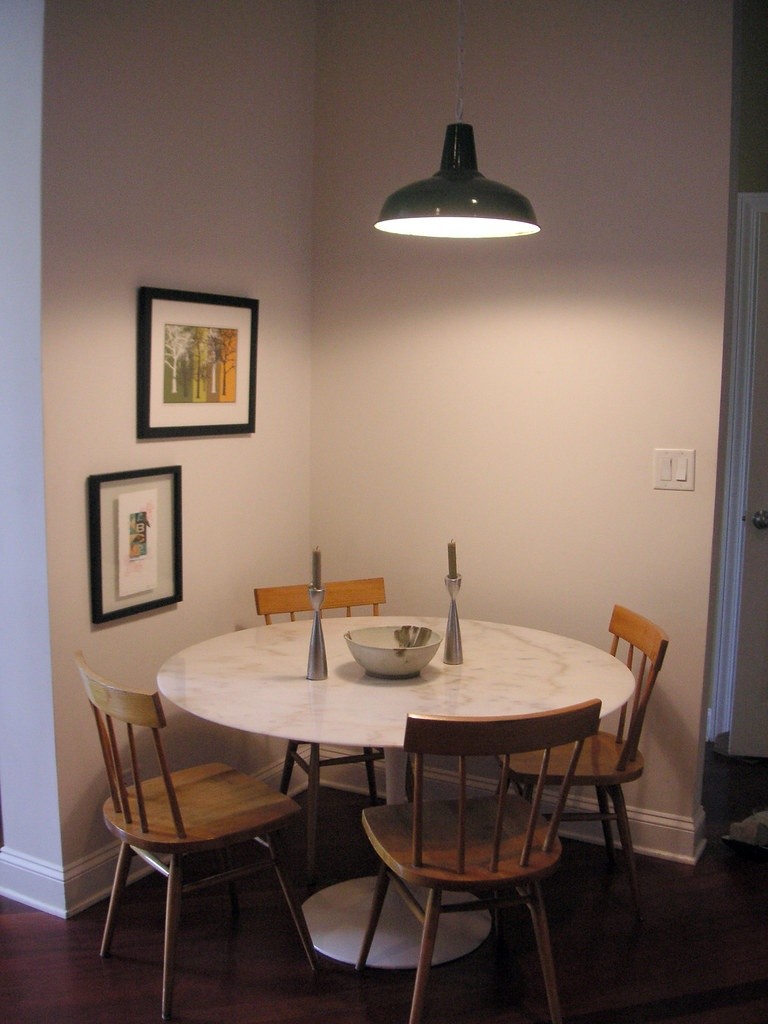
[344,625,443,678]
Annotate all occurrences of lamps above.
[374,1,543,237]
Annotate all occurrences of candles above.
[311,545,320,589]
[449,539,458,578]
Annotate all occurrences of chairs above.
[500,604,670,910]
[353,699,605,1024]
[71,648,322,1022]
[255,576,415,869]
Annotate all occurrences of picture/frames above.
[138,286,259,437]
[86,464,183,624]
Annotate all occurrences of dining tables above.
[157,616,637,969]
